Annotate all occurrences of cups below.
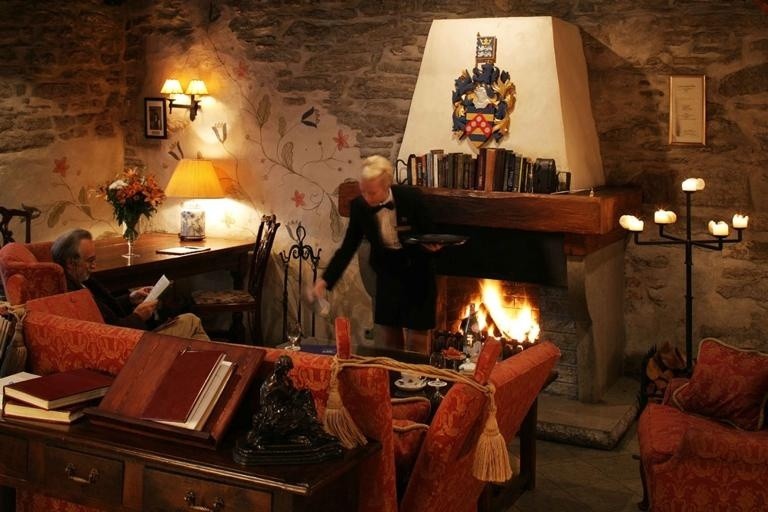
[457,357,476,377]
[402,369,427,384]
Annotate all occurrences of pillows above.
[673,333,767,434]
[388,395,430,417]
[389,419,430,458]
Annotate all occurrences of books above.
[407,148,571,195]
[1,368,116,425]
[140,350,235,432]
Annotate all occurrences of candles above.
[619,177,749,236]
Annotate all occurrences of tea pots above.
[307,287,331,318]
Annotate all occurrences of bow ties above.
[371,201,395,213]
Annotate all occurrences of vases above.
[118,221,140,259]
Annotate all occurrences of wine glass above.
[284,323,303,352]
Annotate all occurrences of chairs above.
[188,216,280,344]
[0,241,67,304]
[637,375,764,512]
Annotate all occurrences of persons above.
[50,229,211,341]
[311,155,444,360]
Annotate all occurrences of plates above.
[393,378,426,391]
[402,234,468,245]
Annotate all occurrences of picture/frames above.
[668,75,706,148]
[142,98,169,140]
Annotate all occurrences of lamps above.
[158,79,208,120]
[162,158,225,240]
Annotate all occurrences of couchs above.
[22,290,556,512]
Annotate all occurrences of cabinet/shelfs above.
[0,414,383,512]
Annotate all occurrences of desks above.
[278,337,556,512]
[8,232,257,342]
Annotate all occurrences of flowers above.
[89,166,168,239]
